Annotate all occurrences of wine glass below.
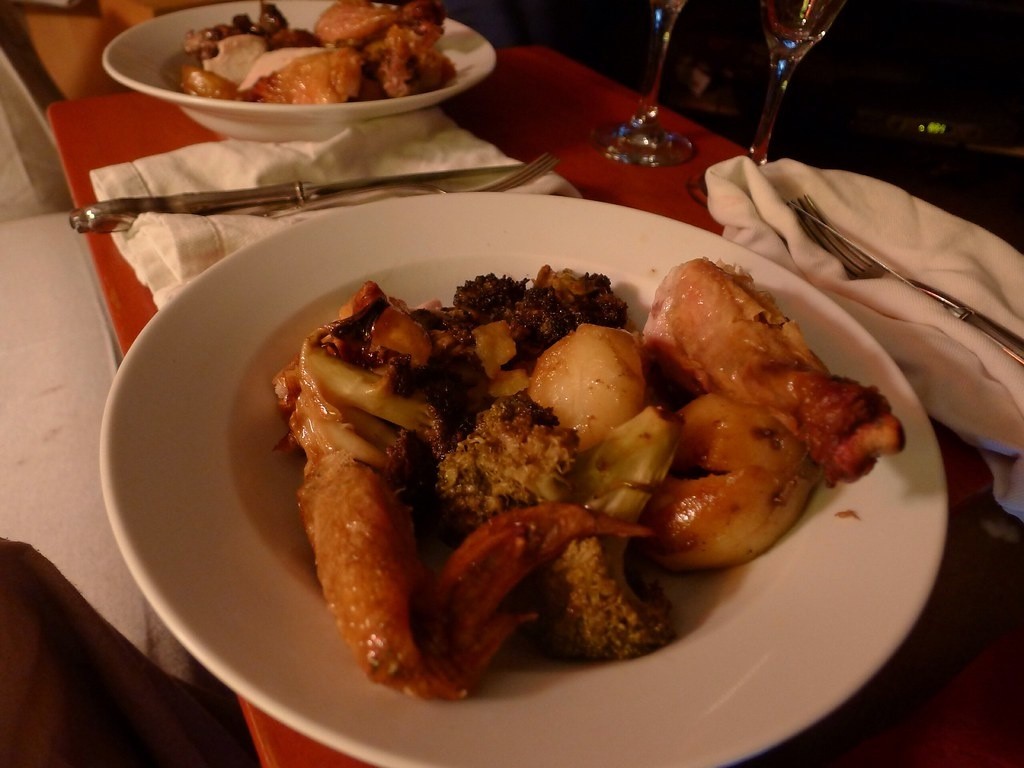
[687,0,847,209]
[590,0,694,167]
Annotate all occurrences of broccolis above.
[421,265,688,661]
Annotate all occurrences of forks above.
[69,154,561,231]
[790,194,1024,366]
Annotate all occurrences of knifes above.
[779,196,1024,374]
[69,165,551,232]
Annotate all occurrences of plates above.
[100,192,947,768]
[102,0,497,142]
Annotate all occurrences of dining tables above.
[45,45,1024,768]
